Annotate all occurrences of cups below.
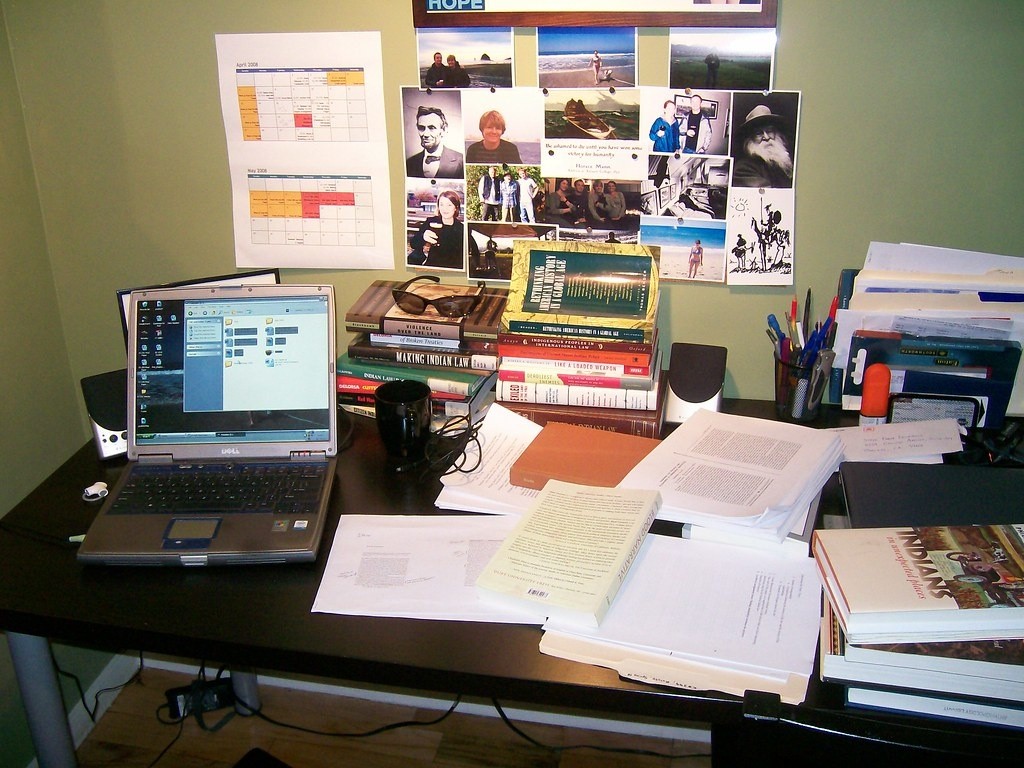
[373,379,432,468]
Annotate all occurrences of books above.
[329,239,1024,730]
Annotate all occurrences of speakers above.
[79,369,130,460]
[666,342,728,426]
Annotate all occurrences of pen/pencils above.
[764,285,840,418]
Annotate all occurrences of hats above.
[740,105,787,136]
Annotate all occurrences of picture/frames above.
[675,94,718,119]
[641,191,658,216]
[659,186,670,209]
[723,108,730,138]
[670,183,676,199]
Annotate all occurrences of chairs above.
[712,690,1023,767]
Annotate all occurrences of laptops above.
[76,284,339,567]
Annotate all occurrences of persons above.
[402,46,796,279]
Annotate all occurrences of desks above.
[0,399,1023,768]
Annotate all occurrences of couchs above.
[666,188,714,219]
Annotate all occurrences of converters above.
[165,678,236,720]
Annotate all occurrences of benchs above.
[569,115,597,127]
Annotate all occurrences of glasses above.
[392,272,485,318]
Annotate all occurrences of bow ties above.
[425,156,441,164]
